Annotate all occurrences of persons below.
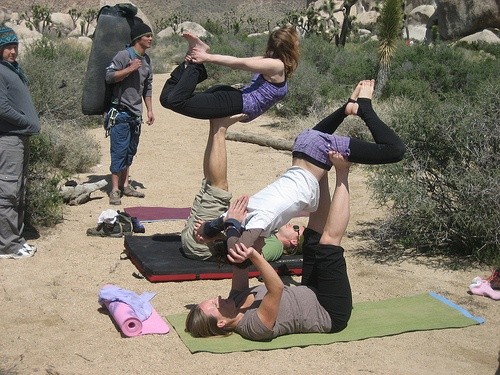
[159,27,300,123]
[182,114,305,260]
[0,25,41,259]
[196,79,407,259]
[185,151,353,341]
[105,24,155,206]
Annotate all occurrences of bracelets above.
[227,225,239,235]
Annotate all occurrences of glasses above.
[293,225,300,241]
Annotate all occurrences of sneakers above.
[87,210,145,237]
[0,243,37,259]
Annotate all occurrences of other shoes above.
[110,190,121,205]
[119,184,144,198]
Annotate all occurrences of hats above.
[130,22,152,42]
[0,23,18,47]
